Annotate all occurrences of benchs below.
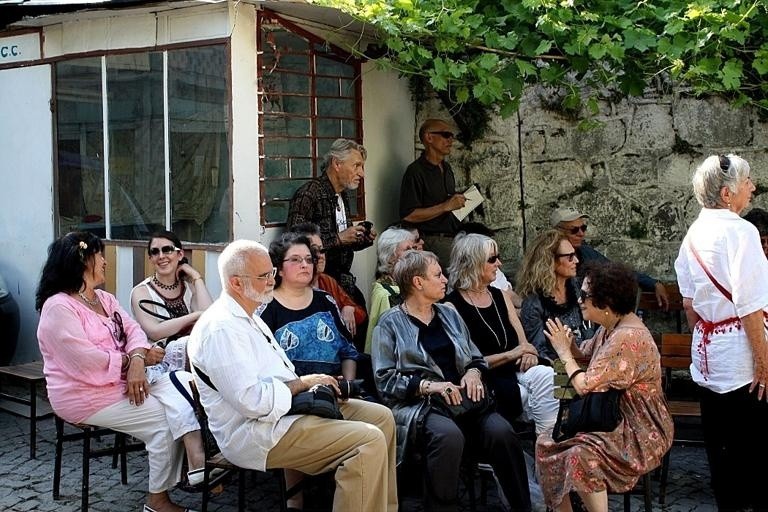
[656,332,707,447]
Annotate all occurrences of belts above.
[420,233,454,238]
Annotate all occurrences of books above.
[452,184,484,224]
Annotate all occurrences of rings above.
[139,383,146,393]
[759,382,766,390]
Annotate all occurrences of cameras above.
[325,379,365,399]
[357,221,373,237]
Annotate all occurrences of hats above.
[550,209,589,227]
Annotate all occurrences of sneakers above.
[187,466,230,486]
[143,503,199,512]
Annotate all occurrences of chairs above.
[553,356,670,512]
[188,380,325,512]
[52,411,147,511]
[637,283,686,311]
[0,361,55,460]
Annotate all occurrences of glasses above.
[112,312,123,341]
[245,268,276,281]
[488,253,500,263]
[281,255,313,265]
[316,248,328,255]
[429,131,455,138]
[558,253,577,262]
[720,154,730,182]
[557,224,587,234]
[150,245,181,255]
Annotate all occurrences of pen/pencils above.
[447,194,472,200]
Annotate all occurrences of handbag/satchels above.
[430,380,496,419]
[492,448,547,512]
[166,314,196,343]
[292,383,340,419]
[552,387,626,443]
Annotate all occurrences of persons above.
[552,208,671,313]
[674,149,768,512]
[399,118,483,273]
[186,223,601,512]
[287,138,375,307]
[129,228,213,370]
[534,257,674,511]
[35,229,232,511]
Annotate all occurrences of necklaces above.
[77,290,100,307]
[151,275,181,290]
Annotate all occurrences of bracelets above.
[122,353,130,371]
[130,351,145,360]
